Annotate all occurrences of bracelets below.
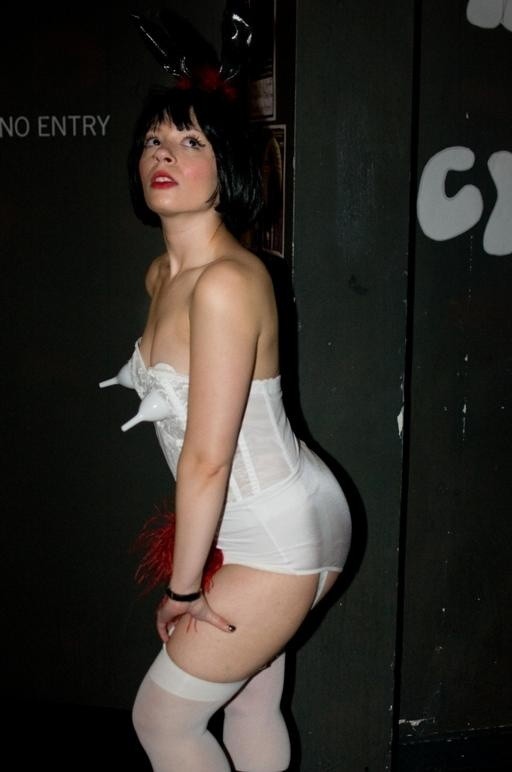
[164,586,203,602]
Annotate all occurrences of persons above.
[96,87,353,772]
